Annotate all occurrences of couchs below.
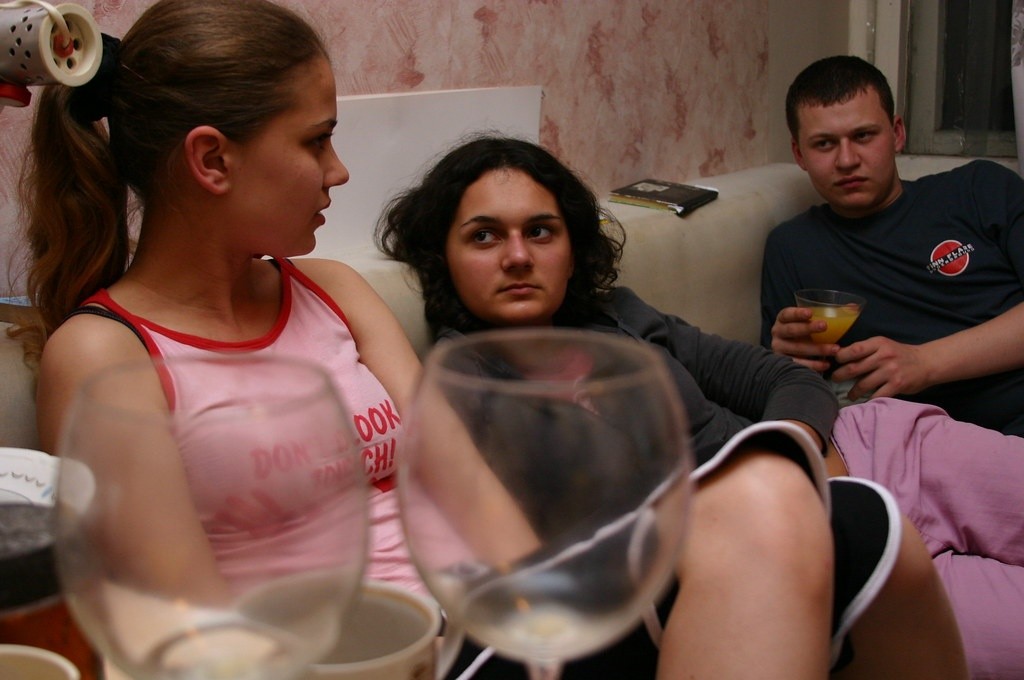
[0,161,829,451]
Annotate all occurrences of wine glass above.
[393,331,692,679]
[794,288,867,380]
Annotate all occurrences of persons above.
[381,137,1024,680]
[762,55,1024,435]
[12,0,965,680]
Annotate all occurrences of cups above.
[52,357,368,680]
[234,575,440,680]
[0,642,81,680]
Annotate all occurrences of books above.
[608,180,719,219]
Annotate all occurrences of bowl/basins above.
[0,444,98,602]
